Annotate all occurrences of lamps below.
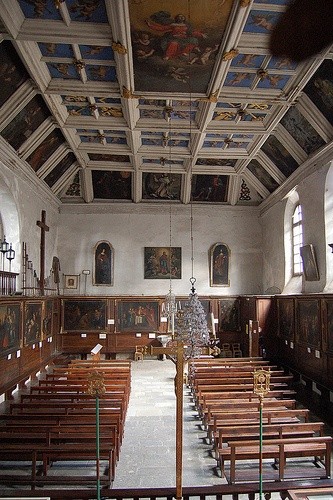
[6,243,15,272]
[0,235,8,271]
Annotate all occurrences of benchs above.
[188,355,333,486]
[0,359,131,490]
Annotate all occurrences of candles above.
[211,312,215,335]
[171,313,175,335]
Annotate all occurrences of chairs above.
[232,343,242,358]
[134,345,145,361]
[223,343,232,357]
